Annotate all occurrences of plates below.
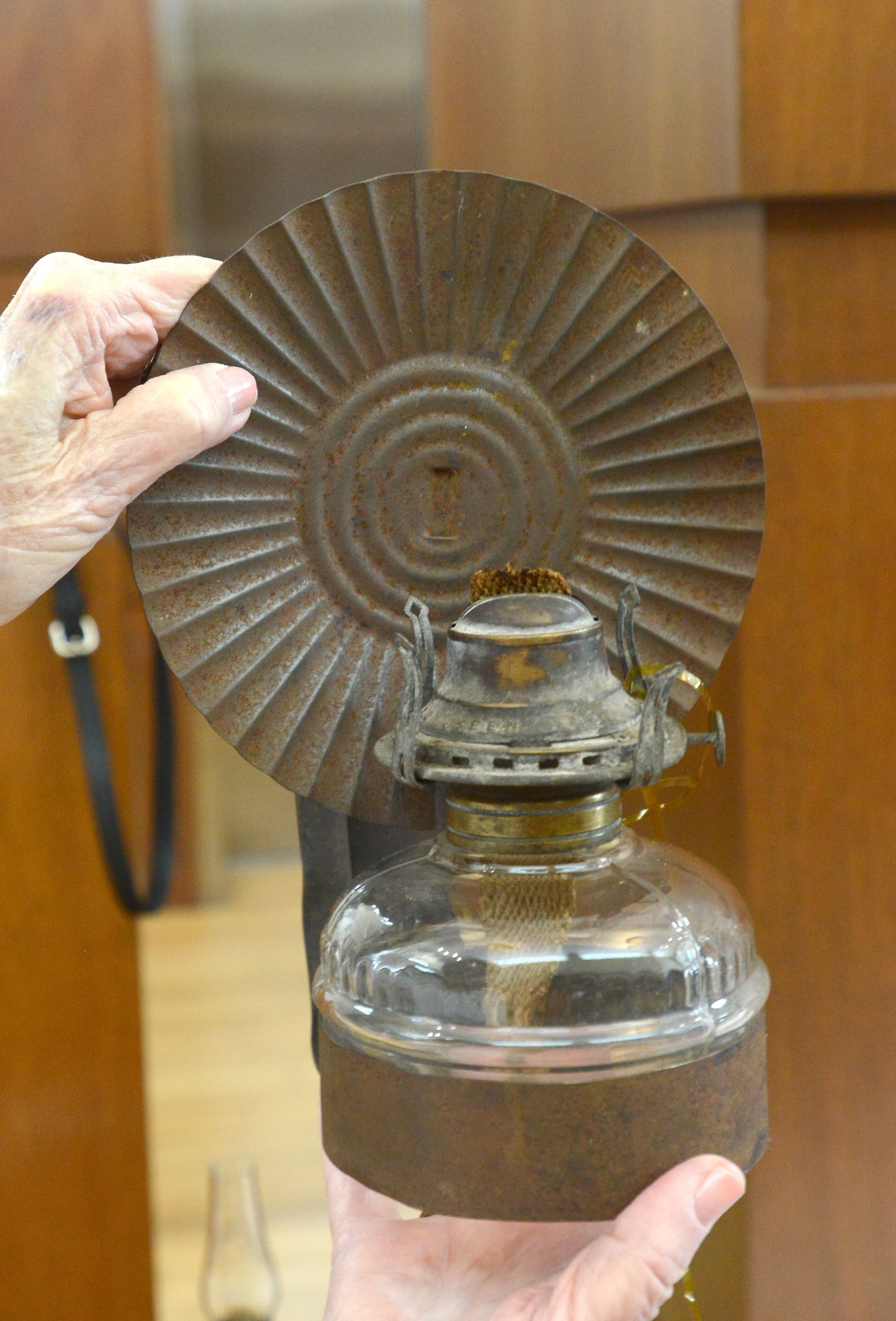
[125,166,768,835]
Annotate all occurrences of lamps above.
[308,551,770,1220]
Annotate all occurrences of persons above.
[0,254,750,1321]
